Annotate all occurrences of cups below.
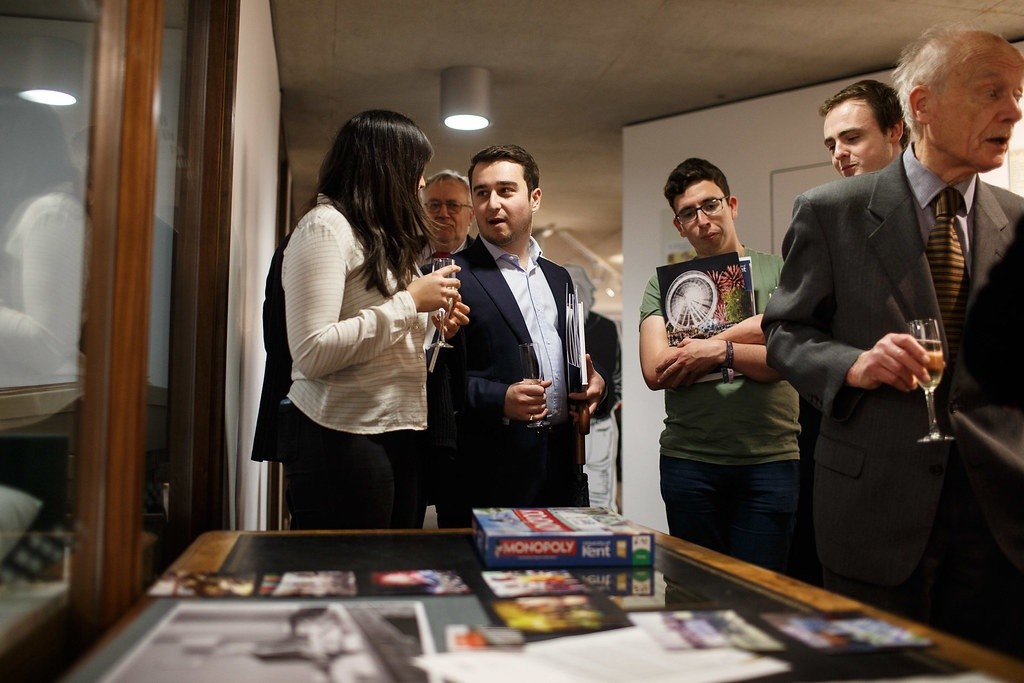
[757,253,784,309]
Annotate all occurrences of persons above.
[431,146,603,509]
[285,110,469,532]
[817,76,908,177]
[641,158,799,578]
[762,23,1024,642]
[398,167,478,529]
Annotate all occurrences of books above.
[560,293,595,424]
[653,249,761,366]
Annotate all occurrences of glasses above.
[423,202,474,214]
[675,195,730,224]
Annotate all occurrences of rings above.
[529,414,534,421]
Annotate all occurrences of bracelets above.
[722,341,737,384]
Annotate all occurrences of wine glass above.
[905,318,956,443]
[427,258,456,349]
[518,343,551,429]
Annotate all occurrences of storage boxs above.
[471,507,657,568]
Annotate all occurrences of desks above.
[56,530,1023,683]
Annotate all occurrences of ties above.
[925,187,964,369]
